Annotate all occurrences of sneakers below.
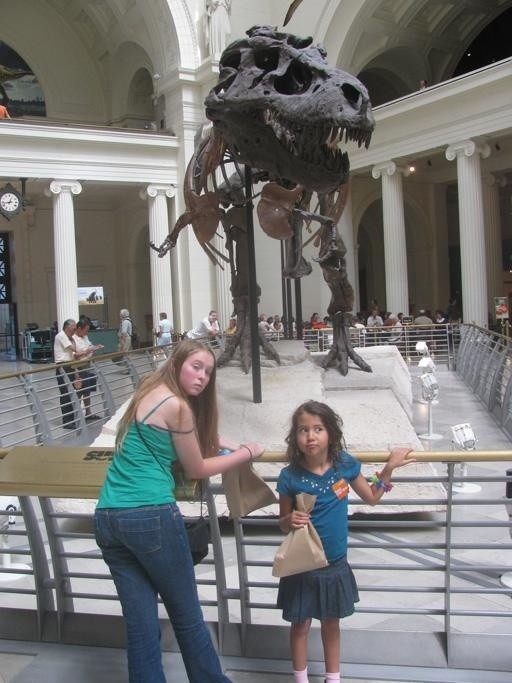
[64,423,77,429]
[120,370,130,375]
[86,414,102,420]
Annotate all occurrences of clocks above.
[0,183,31,221]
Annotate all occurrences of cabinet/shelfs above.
[22,329,53,363]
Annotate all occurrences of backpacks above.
[124,319,137,340]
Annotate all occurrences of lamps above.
[447,423,482,493]
[416,342,444,441]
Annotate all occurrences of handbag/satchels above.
[89,373,96,391]
[184,515,210,566]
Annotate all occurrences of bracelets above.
[369,469,395,493]
[244,445,254,462]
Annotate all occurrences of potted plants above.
[169,328,179,342]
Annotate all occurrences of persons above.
[419,80,429,91]
[350,298,461,356]
[116,308,237,373]
[91,339,267,683]
[53,314,104,430]
[272,399,418,682]
[257,313,332,353]
[0,104,11,119]
